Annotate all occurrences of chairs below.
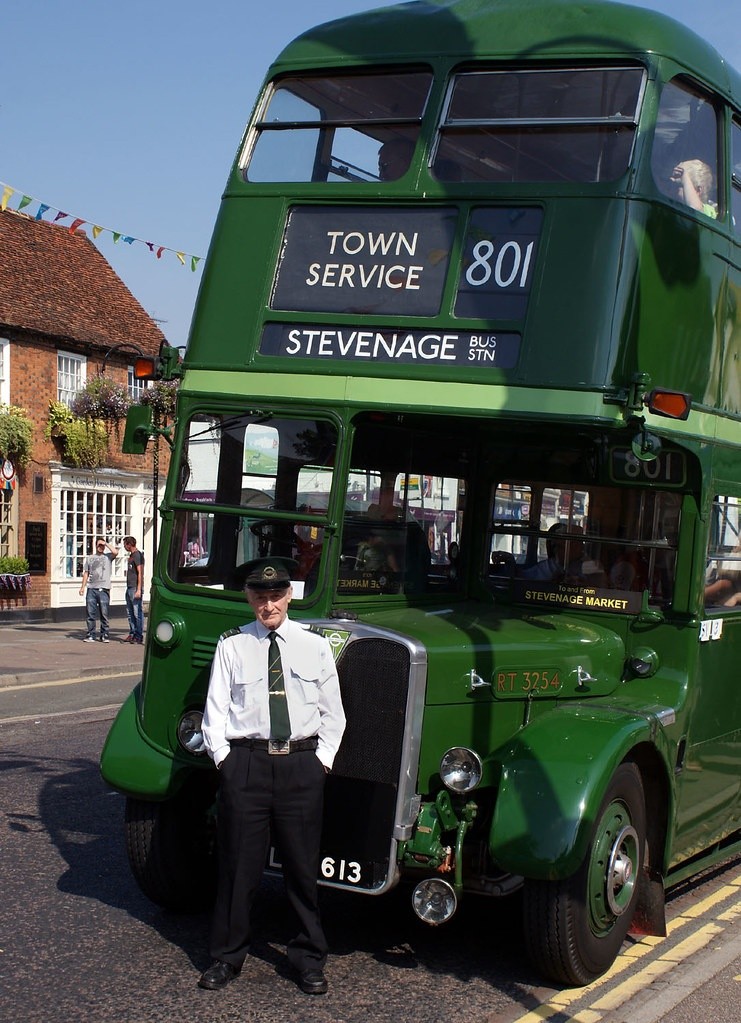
[446,542,458,584]
[492,550,516,577]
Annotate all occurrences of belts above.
[230,739,317,754]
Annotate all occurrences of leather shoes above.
[301,971,328,996]
[198,961,241,990]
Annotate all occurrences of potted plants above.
[0,403,35,468]
[48,402,110,471]
[0,556,30,589]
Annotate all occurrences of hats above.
[236,557,300,593]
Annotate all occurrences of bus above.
[97,0,741,987]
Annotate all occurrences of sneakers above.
[99,634,110,643]
[82,633,95,643]
[120,636,132,644]
[132,639,143,646]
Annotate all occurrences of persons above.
[123,537,145,644]
[79,538,118,642]
[190,537,198,556]
[612,526,667,596]
[379,139,415,182]
[671,159,719,219]
[526,525,608,588]
[199,556,346,990]
[705,579,741,607]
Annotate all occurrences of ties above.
[266,631,293,752]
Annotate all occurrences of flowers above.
[140,378,182,416]
[75,376,128,418]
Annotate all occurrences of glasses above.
[377,160,395,168]
